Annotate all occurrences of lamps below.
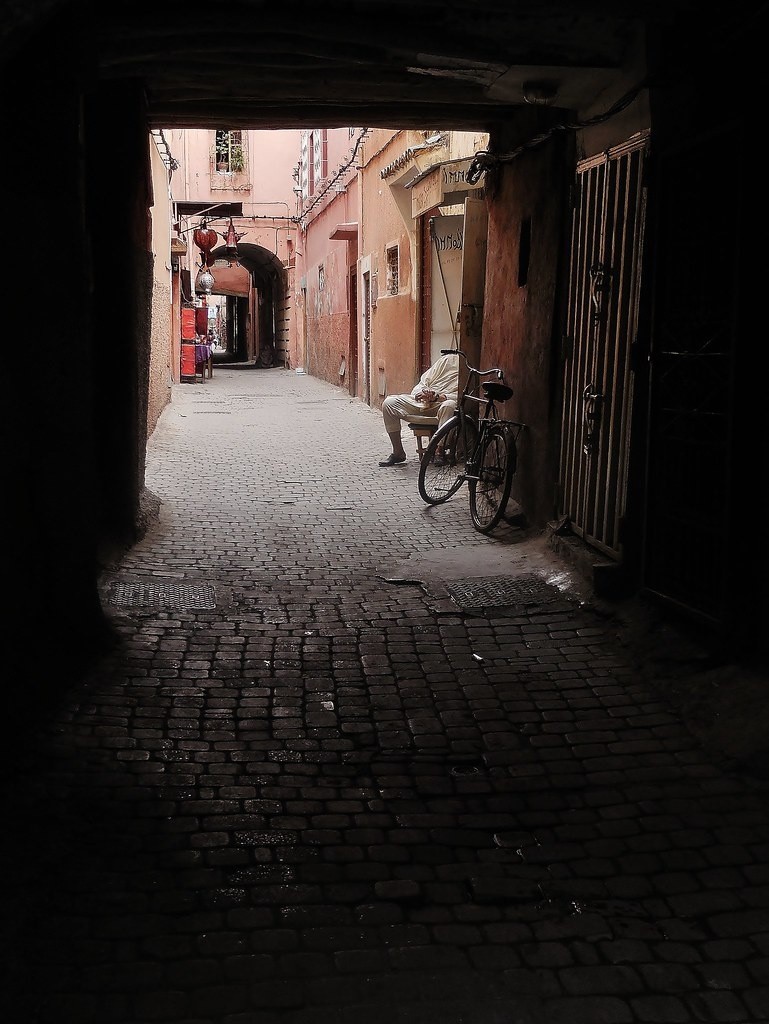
[335,184,347,193]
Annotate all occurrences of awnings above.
[173,201,244,219]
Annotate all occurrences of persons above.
[378,352,459,468]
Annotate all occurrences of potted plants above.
[213,130,244,172]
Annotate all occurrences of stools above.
[407,423,457,465]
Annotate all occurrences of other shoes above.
[435,458,446,465]
[379,453,406,466]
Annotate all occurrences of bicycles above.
[417,349,529,533]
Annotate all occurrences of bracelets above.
[432,394,439,402]
